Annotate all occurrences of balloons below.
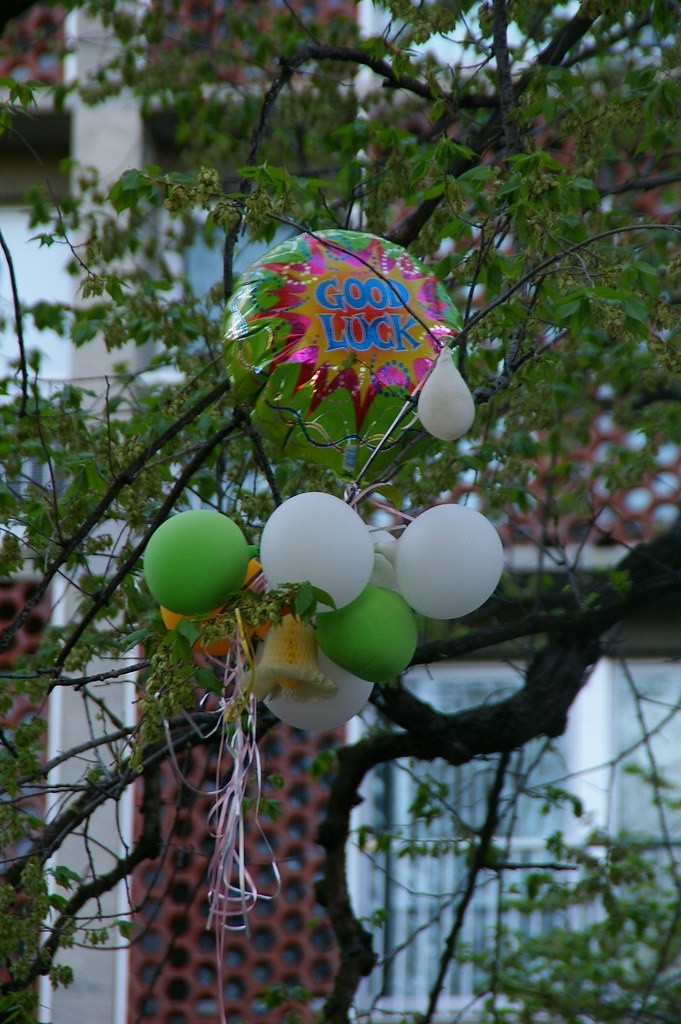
[221,230,464,483]
[419,347,475,442]
[142,493,421,732]
[396,502,505,619]
